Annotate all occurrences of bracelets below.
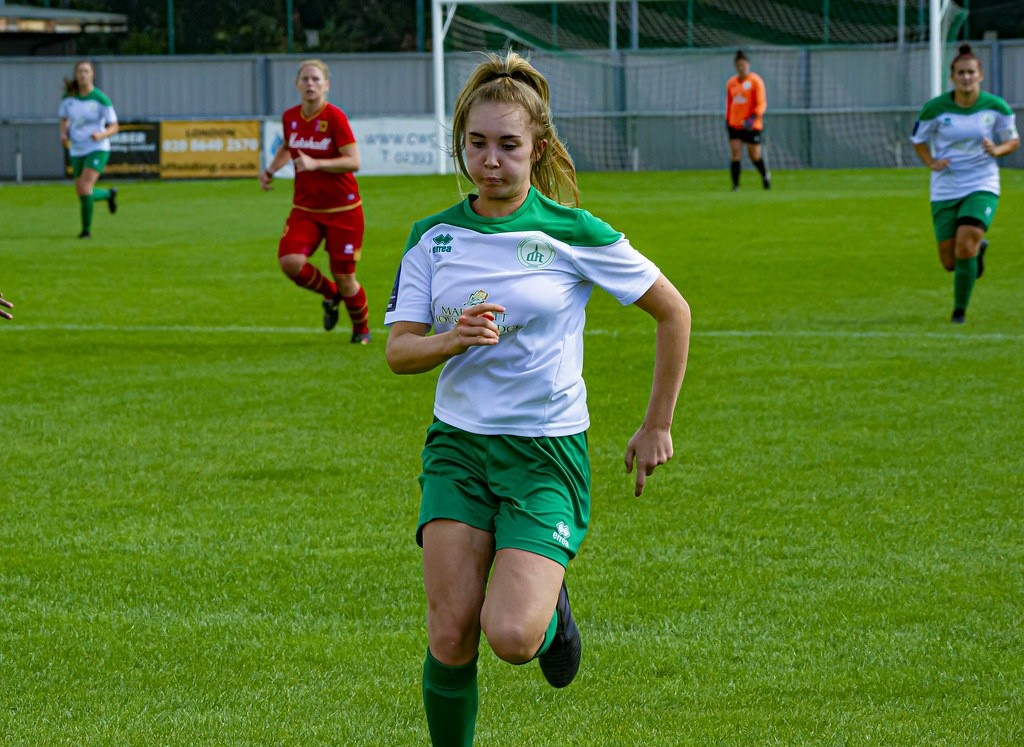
[266,170,273,178]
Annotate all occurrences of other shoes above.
[976,240,987,279]
[761,174,770,187]
[78,231,91,239]
[951,307,964,321]
[107,188,118,214]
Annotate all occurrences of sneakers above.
[538,577,581,689]
[322,292,342,330]
[350,333,371,345]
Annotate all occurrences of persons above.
[910,44,1021,323]
[259,59,370,345]
[723,49,771,191]
[383,48,692,747]
[58,60,118,240]
